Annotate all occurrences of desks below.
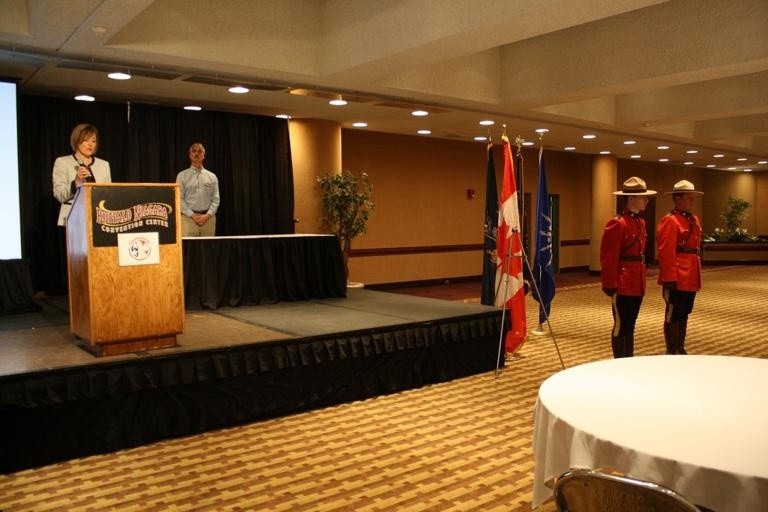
[527,353,766,511]
[185,231,353,313]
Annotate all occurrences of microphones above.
[78,160,90,182]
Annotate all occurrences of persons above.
[597,174,657,359]
[49,123,115,229]
[173,142,223,238]
[655,179,706,355]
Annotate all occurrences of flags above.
[480,139,500,306]
[529,146,557,325]
[512,151,533,297]
[491,136,526,357]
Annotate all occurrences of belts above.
[678,247,704,254]
[193,210,208,214]
[619,254,645,261]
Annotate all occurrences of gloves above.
[663,281,675,290]
[603,288,616,296]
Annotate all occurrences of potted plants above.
[313,170,374,292]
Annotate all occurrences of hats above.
[612,176,658,197]
[664,179,704,196]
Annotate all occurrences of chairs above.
[552,467,705,512]
